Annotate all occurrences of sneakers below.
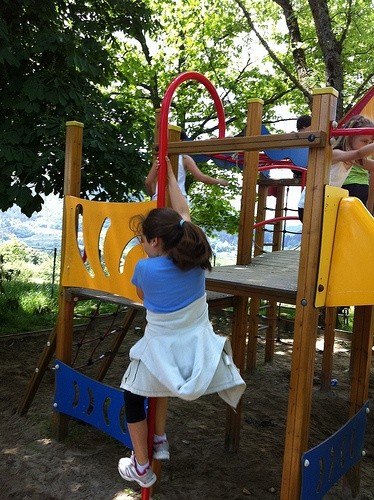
[152,439,170,460]
[117,450,157,488]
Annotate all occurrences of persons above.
[341,156,369,207]
[299,115,374,225]
[118,208,246,489]
[145,130,229,207]
[297,116,311,132]
